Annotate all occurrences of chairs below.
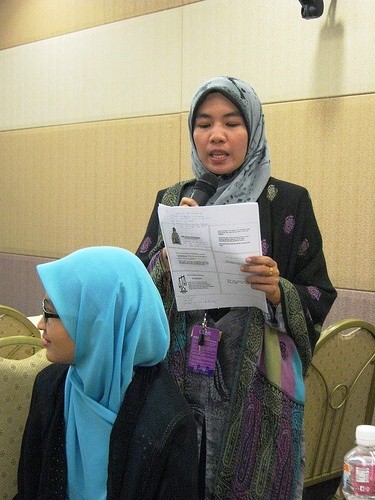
[303,319,374,500]
[0,306,60,500]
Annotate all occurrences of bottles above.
[343,425,374,500]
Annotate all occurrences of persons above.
[134,75,338,500]
[13,247,201,500]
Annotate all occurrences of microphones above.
[186,172,219,207]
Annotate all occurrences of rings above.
[268,266,273,275]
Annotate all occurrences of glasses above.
[42,298,60,323]
